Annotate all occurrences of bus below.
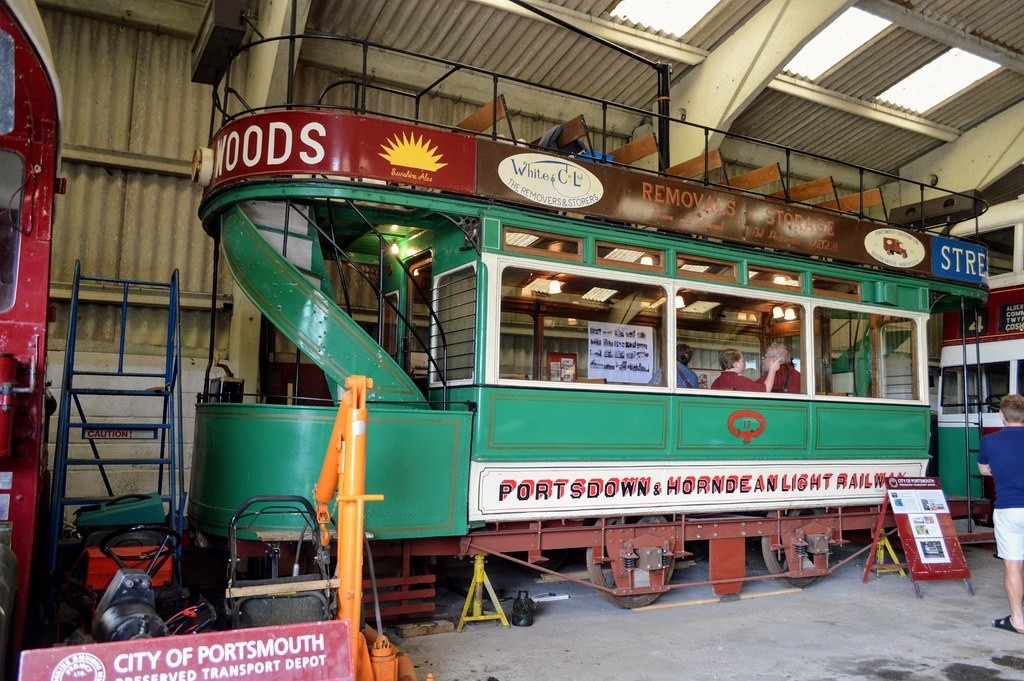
[0,0,66,681]
[184,34,989,607]
[935,271,1024,520]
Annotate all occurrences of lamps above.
[549,253,797,321]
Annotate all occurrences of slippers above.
[992,614,1024,634]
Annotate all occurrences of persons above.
[648,343,699,389]
[710,349,782,392]
[977,394,1024,634]
[755,342,800,394]
